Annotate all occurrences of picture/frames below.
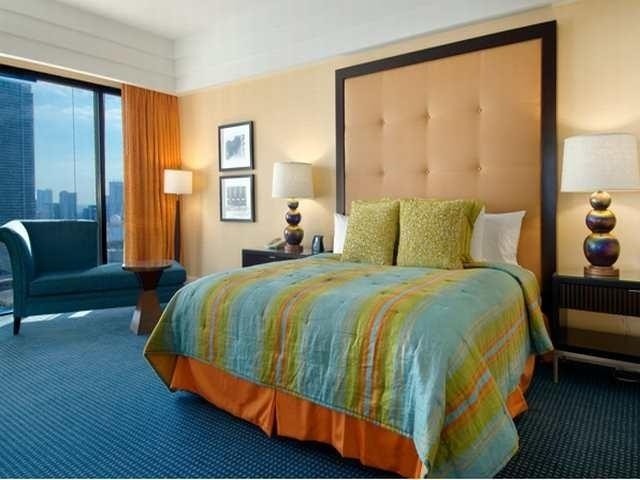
[216,120,256,222]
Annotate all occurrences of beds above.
[182,251,535,479]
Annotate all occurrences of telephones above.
[265,238,287,250]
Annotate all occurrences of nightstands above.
[545,272,639,386]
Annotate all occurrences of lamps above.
[163,167,193,262]
[271,160,317,253]
[559,133,640,275]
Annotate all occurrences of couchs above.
[1,216,189,335]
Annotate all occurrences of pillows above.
[331,196,531,276]
